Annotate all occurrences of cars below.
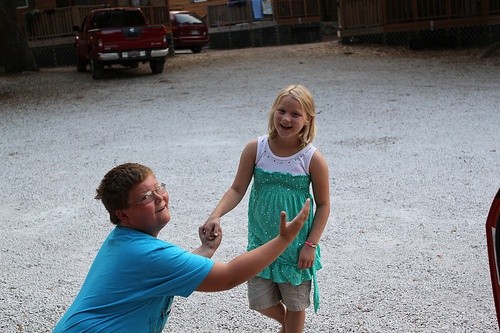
[169,11,208,53]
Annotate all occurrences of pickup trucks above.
[73,8,170,79]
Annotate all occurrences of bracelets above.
[304,241,317,249]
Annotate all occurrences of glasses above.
[128,182,166,214]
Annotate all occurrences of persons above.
[52,163,311,333]
[204,84,330,333]
